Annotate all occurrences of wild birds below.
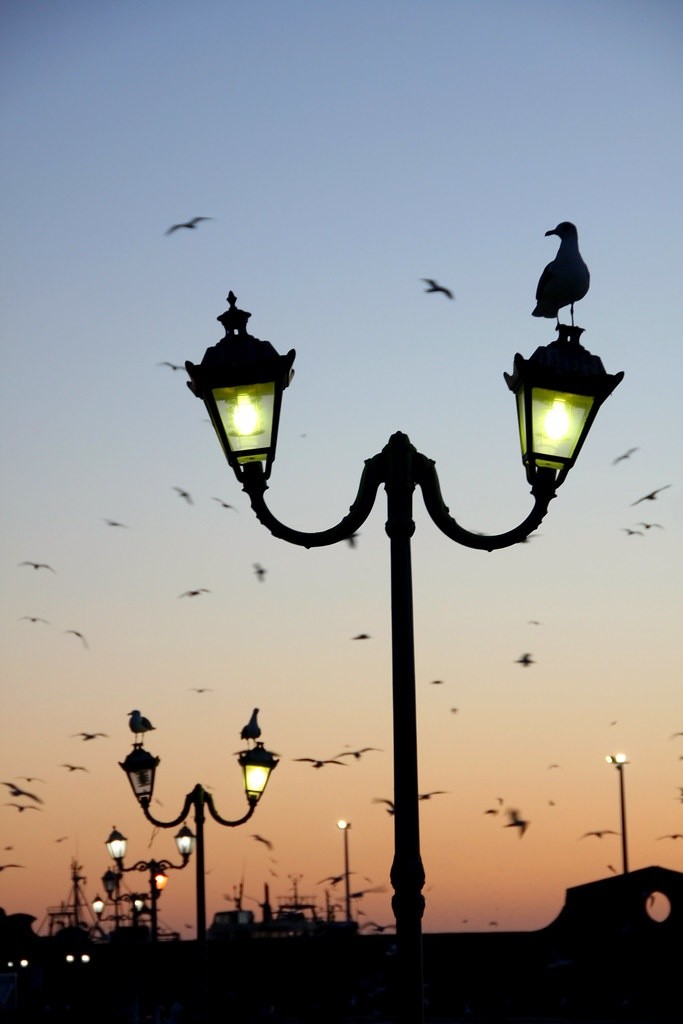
[12,560,92,654]
[292,747,388,768]
[373,791,449,815]
[427,651,542,718]
[248,833,274,851]
[420,276,455,300]
[99,485,242,528]
[164,216,215,235]
[315,871,376,901]
[0,732,110,870]
[531,221,591,330]
[177,562,271,600]
[191,687,211,694]
[610,446,673,535]
[480,797,620,874]
[162,359,189,372]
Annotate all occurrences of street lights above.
[184,289,626,1024]
[338,821,351,921]
[106,821,196,935]
[91,893,145,930]
[606,753,629,872]
[119,742,279,1024]
[102,866,170,928]
